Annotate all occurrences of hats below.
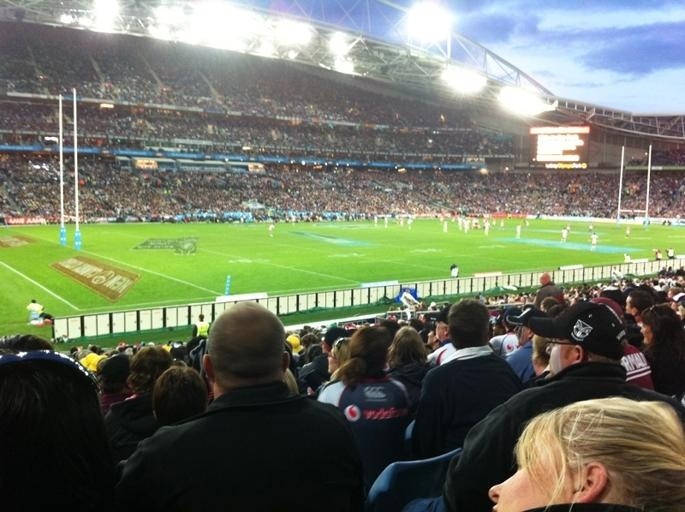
[425,304,453,324]
[528,301,617,346]
[506,306,547,328]
[663,291,684,304]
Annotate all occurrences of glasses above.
[543,337,575,345]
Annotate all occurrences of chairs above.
[367,443,469,511]
